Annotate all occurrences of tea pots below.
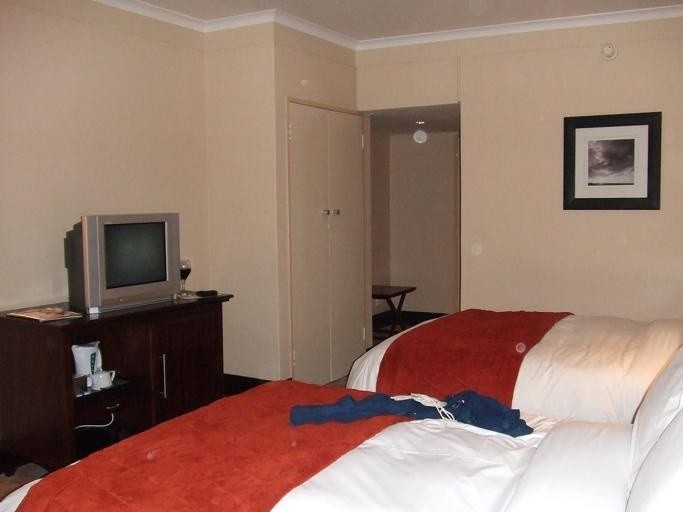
[89,368,116,391]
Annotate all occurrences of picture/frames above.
[564,112,661,210]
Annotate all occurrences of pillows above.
[633,347,681,474]
[623,409,680,510]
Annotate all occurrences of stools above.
[372,284,417,339]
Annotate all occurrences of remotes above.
[196,290,217,297]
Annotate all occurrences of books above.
[6,306,83,323]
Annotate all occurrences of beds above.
[0,380,632,508]
[346,309,682,425]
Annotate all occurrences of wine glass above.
[177,258,192,296]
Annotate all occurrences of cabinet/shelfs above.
[285,104,368,388]
[1,304,223,475]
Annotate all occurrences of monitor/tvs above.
[64,212,181,314]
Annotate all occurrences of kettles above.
[70,339,104,388]
